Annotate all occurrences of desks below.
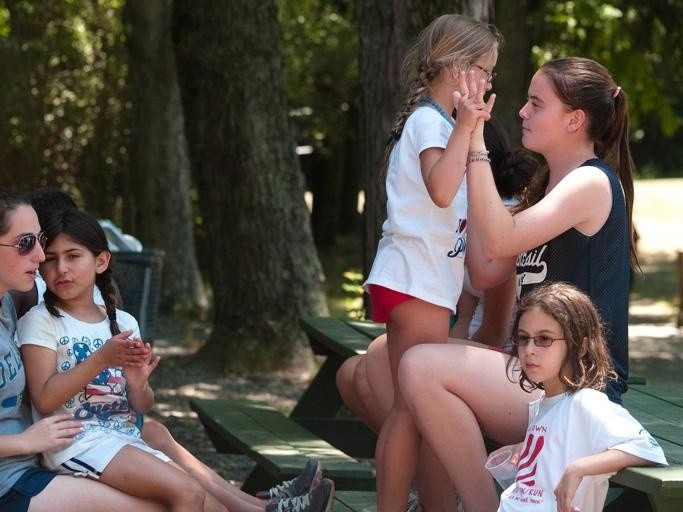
[302,310,683,509]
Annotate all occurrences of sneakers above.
[265,477,335,511]
[256,457,323,500]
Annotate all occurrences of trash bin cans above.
[109,249,166,350]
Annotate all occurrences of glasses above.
[1,231,48,257]
[510,334,566,347]
[476,64,498,83]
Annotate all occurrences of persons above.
[1,189,336,512]
[336,13,669,512]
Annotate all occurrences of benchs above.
[191,393,371,488]
